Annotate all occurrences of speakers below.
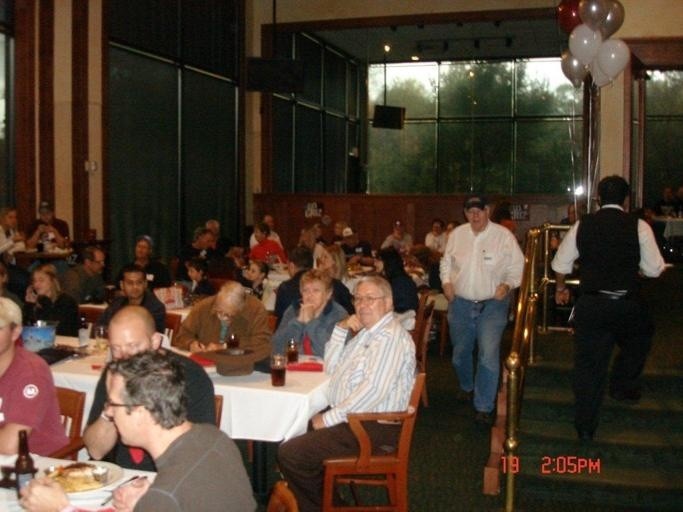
[246,59,304,89]
[373,104,407,131]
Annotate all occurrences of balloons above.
[556,0,632,90]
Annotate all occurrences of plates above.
[58,460,125,493]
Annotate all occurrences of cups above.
[36,243,43,252]
[95,324,107,352]
[225,333,239,349]
[269,352,288,386]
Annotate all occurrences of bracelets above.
[101,412,114,421]
[557,287,566,293]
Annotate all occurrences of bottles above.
[77,316,93,346]
[287,337,297,364]
[16,429,34,500]
[171,282,184,309]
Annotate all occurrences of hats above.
[463,198,487,209]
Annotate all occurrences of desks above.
[49,341,331,493]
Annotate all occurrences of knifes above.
[100,476,146,506]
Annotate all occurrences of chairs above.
[49,278,448,512]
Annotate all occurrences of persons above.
[552,175,666,440]
[280,276,419,512]
[642,185,682,241]
[83,305,216,473]
[18,348,258,512]
[494,201,515,234]
[0,298,72,460]
[0,198,461,368]
[550,203,580,249]
[440,192,523,425]
